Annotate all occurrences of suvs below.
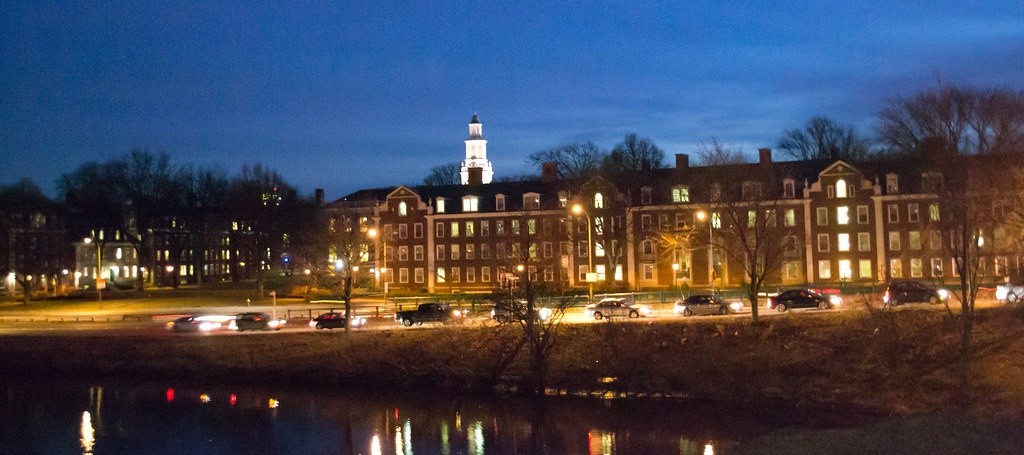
[491,299,549,324]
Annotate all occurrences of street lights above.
[573,203,593,304]
[695,209,714,286]
[84,236,101,306]
[367,227,387,293]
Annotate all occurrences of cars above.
[996,280,1024,303]
[674,294,743,317]
[586,298,651,320]
[228,311,272,332]
[310,312,363,330]
[883,279,950,307]
[167,315,208,333]
[767,288,841,313]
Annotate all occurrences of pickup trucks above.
[394,302,463,326]
